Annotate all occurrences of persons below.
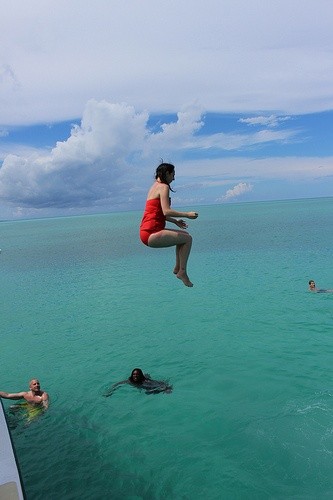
[139,157,199,287]
[309,280,320,291]
[125,368,149,383]
[0,378,49,408]
